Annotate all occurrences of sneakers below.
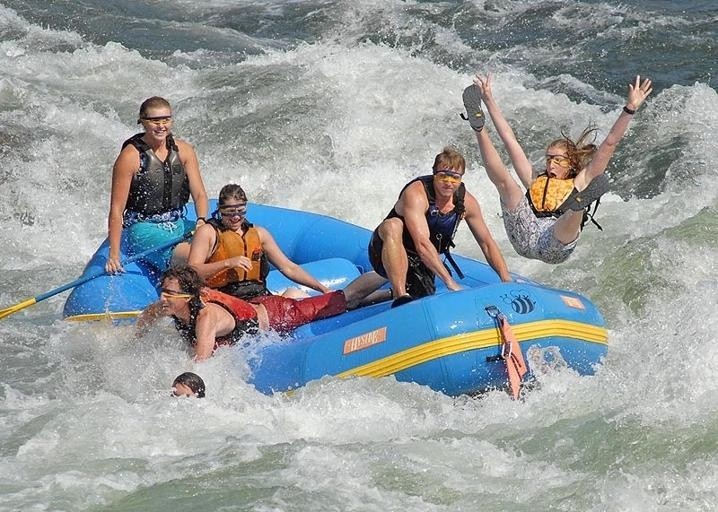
[463,84,486,131]
[391,294,414,309]
[568,174,610,212]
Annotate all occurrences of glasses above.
[217,201,248,216]
[545,154,572,169]
[158,286,189,296]
[434,170,462,183]
[141,116,173,125]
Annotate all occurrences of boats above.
[62,197,609,403]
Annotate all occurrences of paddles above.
[0,229,201,320]
[62,311,146,320]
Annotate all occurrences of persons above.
[459,70,653,265]
[188,182,332,301]
[170,371,207,399]
[137,265,413,364]
[365,147,514,308]
[102,94,208,275]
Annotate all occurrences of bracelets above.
[620,106,637,115]
[196,216,207,223]
